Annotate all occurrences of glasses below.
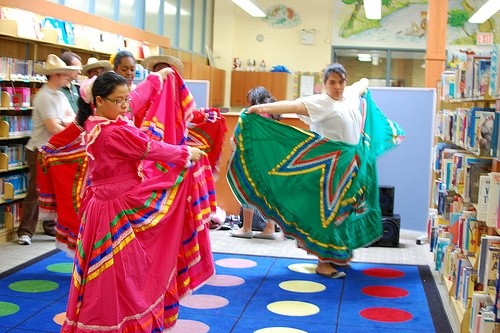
[103,95,133,106]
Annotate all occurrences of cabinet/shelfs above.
[429,94,500,333]
[0,33,144,246]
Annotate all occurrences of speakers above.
[378,185,395,215]
[378,215,400,247]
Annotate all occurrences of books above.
[426,44,500,333]
[0,57,48,230]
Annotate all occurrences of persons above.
[231,85,282,240]
[245,64,370,279]
[16,54,78,245]
[35,52,184,232]
[63,71,203,333]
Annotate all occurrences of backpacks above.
[239,204,282,232]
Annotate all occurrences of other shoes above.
[317,269,347,279]
[19,234,32,245]
[254,232,276,239]
[228,228,253,238]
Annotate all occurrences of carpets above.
[0,248,456,333]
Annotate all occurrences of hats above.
[81,56,112,75]
[34,53,81,75]
[141,55,184,75]
